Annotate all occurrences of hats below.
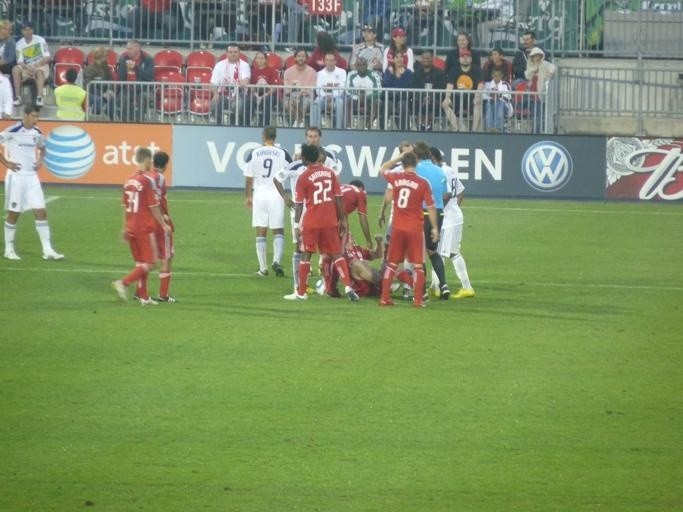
[460,50,471,57]
[362,25,376,32]
[392,28,406,37]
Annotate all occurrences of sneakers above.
[256,269,268,277]
[344,286,360,302]
[272,261,284,277]
[42,248,64,260]
[327,287,340,296]
[380,297,392,305]
[391,283,449,306]
[4,243,20,259]
[113,279,127,299]
[13,96,23,106]
[35,96,44,106]
[134,294,177,305]
[450,287,475,299]
[283,286,308,300]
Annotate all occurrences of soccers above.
[315,280,325,295]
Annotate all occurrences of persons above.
[275,128,477,305]
[108,145,180,306]
[240,123,294,277]
[1,102,68,262]
[1,0,558,133]
